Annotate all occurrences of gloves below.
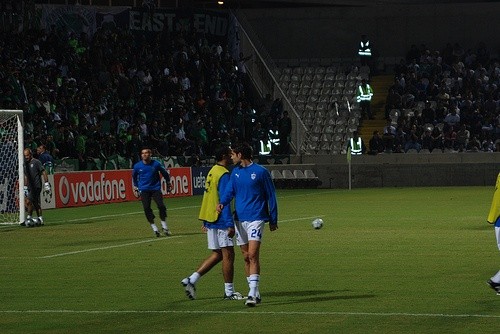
[166,183,172,192]
[133,186,140,198]
[24,186,29,200]
[43,182,51,193]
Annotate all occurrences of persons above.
[0,20,292,175]
[23,148,50,227]
[347,131,367,156]
[367,43,500,156]
[132,147,174,237]
[180,146,248,300]
[216,141,279,306]
[358,79,374,120]
[485,172,500,295]
[354,33,377,77]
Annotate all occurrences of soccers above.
[312,219,323,230]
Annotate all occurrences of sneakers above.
[486,279,500,295]
[247,294,256,307]
[155,231,160,238]
[28,218,35,226]
[223,292,248,300]
[180,277,196,300]
[162,228,172,236]
[255,291,261,304]
[35,218,44,227]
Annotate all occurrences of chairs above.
[279,66,371,155]
[269,169,320,189]
